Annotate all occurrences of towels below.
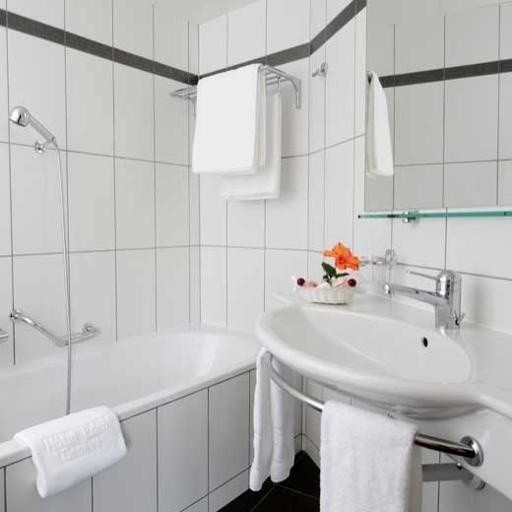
[212,95,281,202]
[13,405,127,498]
[192,62,265,173]
[366,72,393,177]
[318,400,422,511]
[249,344,296,492]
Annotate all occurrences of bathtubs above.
[0,320,302,512]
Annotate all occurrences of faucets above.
[382,269,463,332]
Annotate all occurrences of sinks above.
[255,292,488,422]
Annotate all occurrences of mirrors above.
[364,1,512,208]
